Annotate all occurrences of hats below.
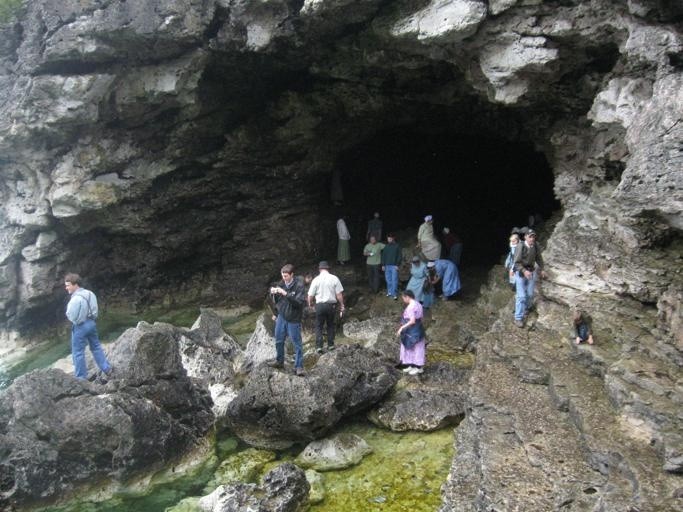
[525,229,537,237]
[318,261,329,269]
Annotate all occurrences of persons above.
[64,273,112,382]
[567,307,595,343]
[395,288,428,376]
[306,260,347,351]
[513,229,546,327]
[335,208,524,305]
[269,264,308,375]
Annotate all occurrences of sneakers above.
[267,360,284,368]
[402,365,412,373]
[408,367,423,375]
[296,366,305,376]
[515,319,525,328]
